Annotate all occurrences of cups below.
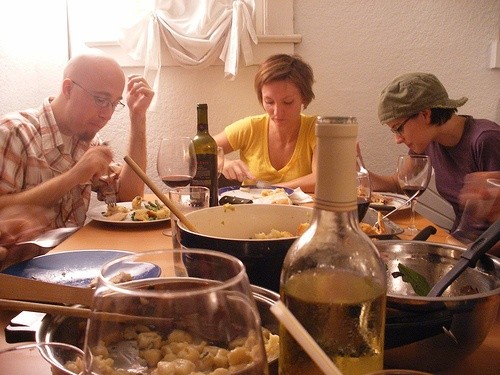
[170,186,210,277]
[355,171,371,224]
[82,248,269,375]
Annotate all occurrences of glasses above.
[391,114,416,134]
[70,80,125,112]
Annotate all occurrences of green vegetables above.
[392,263,430,297]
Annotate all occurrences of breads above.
[262,187,293,204]
[359,211,387,234]
[148,206,170,219]
[359,186,392,207]
[254,223,309,239]
[103,195,147,221]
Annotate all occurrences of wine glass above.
[397,155,432,236]
[157,136,197,236]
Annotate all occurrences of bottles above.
[187,103,218,208]
[277,115,387,375]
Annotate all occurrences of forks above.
[102,143,117,207]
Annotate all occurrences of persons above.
[214,55,318,193]
[0,54,155,256]
[357,73,500,258]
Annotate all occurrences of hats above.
[377,72,468,124]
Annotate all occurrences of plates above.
[359,206,404,240]
[85,201,170,226]
[0,249,161,304]
[368,191,410,210]
[217,185,294,205]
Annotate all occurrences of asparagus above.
[145,200,162,211]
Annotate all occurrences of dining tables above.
[0,187,500,375]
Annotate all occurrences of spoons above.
[0,226,79,248]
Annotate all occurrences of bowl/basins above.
[35,277,280,375]
[369,238,500,371]
[177,203,314,321]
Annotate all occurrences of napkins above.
[288,187,313,204]
